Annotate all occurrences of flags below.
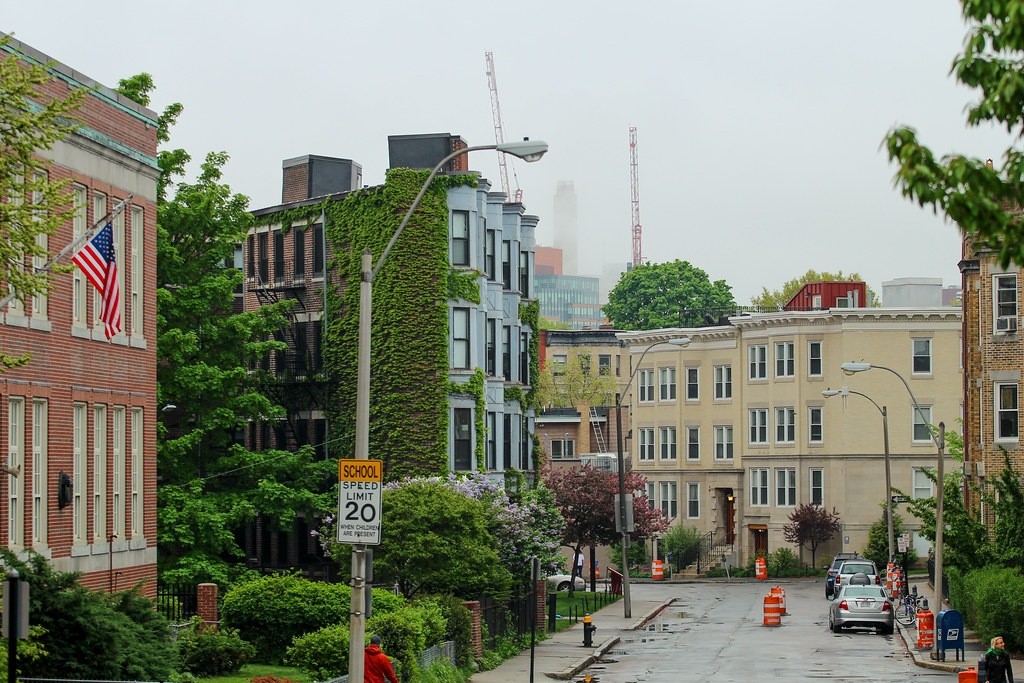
[73,222,121,339]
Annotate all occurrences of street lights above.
[821,387,895,563]
[727,493,735,552]
[616,337,691,618]
[841,362,946,660]
[350,134,552,683]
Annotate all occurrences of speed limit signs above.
[337,458,383,546]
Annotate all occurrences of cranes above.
[628,124,649,270]
[484,50,524,204]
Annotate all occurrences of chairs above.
[863,568,872,575]
[845,567,853,573]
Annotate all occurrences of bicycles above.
[895,591,924,624]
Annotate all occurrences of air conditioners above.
[996,318,1016,331]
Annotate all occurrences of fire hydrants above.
[582,611,597,647]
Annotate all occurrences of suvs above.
[823,550,869,599]
[832,559,883,601]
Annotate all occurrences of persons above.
[985,637,1014,683]
[364,635,398,683]
[573,550,584,578]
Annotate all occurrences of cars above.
[546,566,586,592]
[828,584,895,635]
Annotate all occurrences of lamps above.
[727,494,736,501]
[0,462,21,478]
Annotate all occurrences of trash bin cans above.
[936,609,964,661]
